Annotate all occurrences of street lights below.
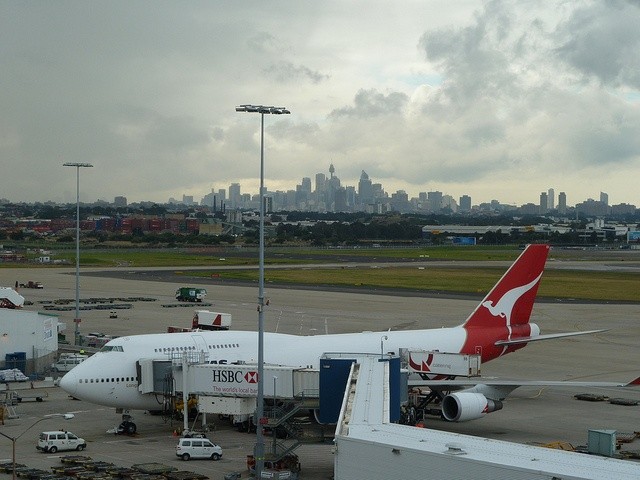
[235,104,291,480]
[62,162,93,346]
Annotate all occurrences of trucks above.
[176,287,207,302]
[192,310,232,330]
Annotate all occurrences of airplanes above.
[56,242,640,439]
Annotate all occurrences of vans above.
[51,359,81,372]
[37,430,87,453]
[176,438,223,460]
